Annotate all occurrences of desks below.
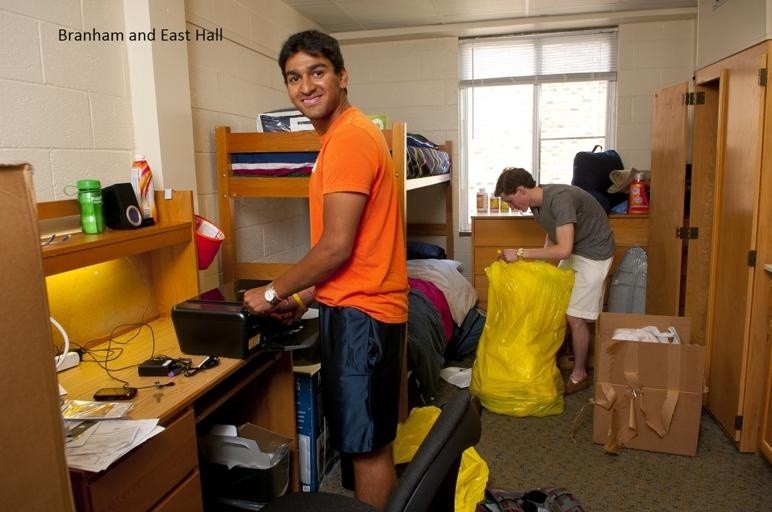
[58,313,297,512]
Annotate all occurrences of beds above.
[214,120,456,422]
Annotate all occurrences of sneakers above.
[564,375,588,395]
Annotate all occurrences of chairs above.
[264,392,482,512]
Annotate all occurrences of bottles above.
[477,188,488,212]
[490,193,499,211]
[131,154,158,224]
[500,198,509,212]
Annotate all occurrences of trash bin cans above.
[195,426,290,512]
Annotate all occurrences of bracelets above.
[288,291,309,317]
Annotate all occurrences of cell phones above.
[94,388,138,400]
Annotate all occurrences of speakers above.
[100,182,145,230]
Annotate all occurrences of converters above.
[139,356,174,378]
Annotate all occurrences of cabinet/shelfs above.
[1,165,201,512]
[472,211,651,307]
[649,39,771,449]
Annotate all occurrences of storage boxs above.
[203,451,290,500]
[593,311,707,458]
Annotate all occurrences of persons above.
[497,168,617,398]
[243,30,410,512]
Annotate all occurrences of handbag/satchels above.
[573,145,629,211]
[477,486,585,512]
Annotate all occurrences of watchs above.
[264,281,282,305]
[516,246,524,263]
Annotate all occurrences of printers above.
[171,278,305,361]
